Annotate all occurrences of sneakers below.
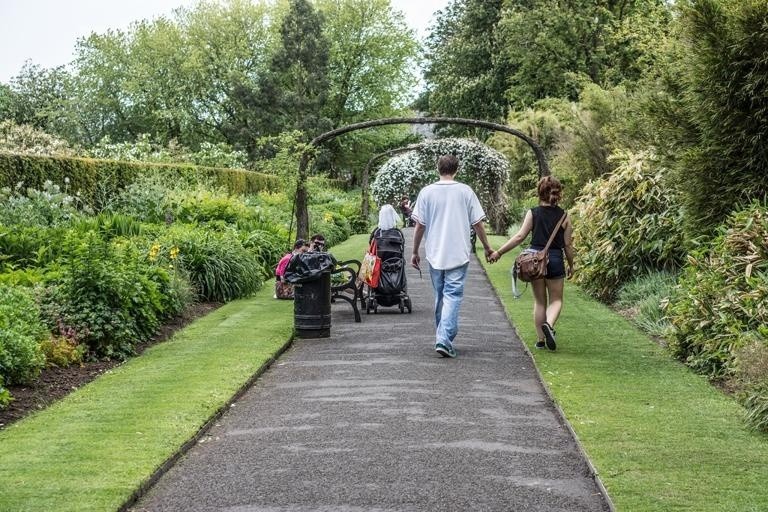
[433,343,456,357]
[535,321,557,350]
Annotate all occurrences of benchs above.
[302,245,363,321]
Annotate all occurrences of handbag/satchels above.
[511,248,549,297]
[275,281,294,299]
[358,238,381,288]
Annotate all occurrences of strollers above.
[364,225,411,314]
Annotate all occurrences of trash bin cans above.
[294,253,330,337]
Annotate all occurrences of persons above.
[276,234,326,300]
[411,155,494,359]
[400,195,416,224]
[489,175,575,352]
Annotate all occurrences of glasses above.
[314,242,325,247]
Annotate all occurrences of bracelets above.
[498,249,504,256]
